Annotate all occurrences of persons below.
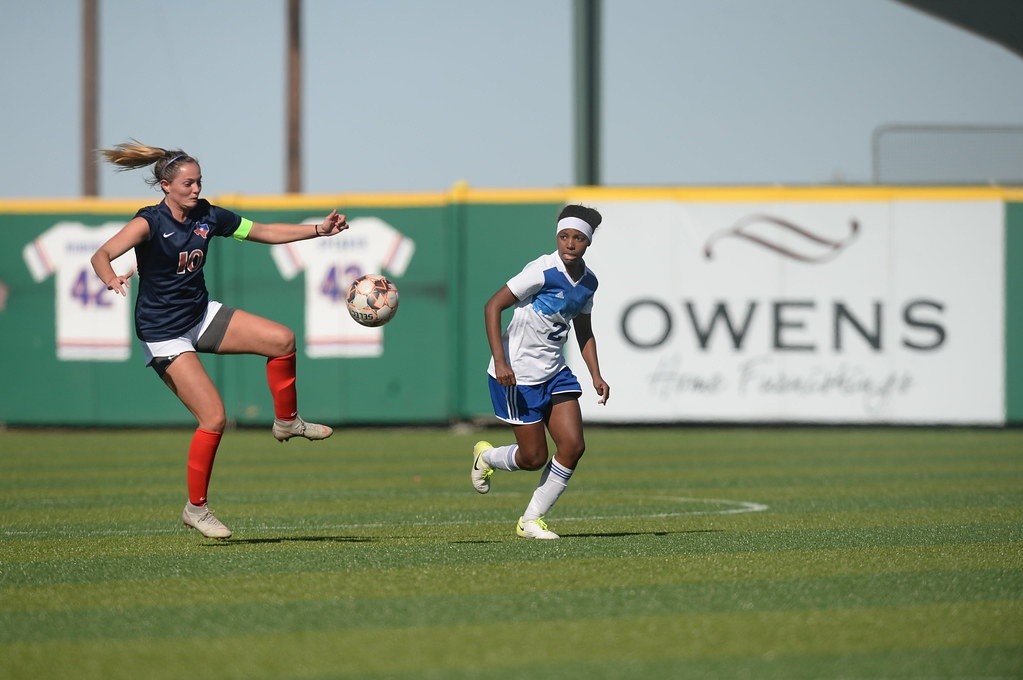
[470,204,610,540]
[90,137,350,541]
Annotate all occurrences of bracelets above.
[315,225,321,236]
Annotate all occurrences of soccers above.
[348,274,398,329]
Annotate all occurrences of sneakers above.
[182,500,232,540]
[273,418,334,443]
[471,441,494,494]
[517,516,560,540]
